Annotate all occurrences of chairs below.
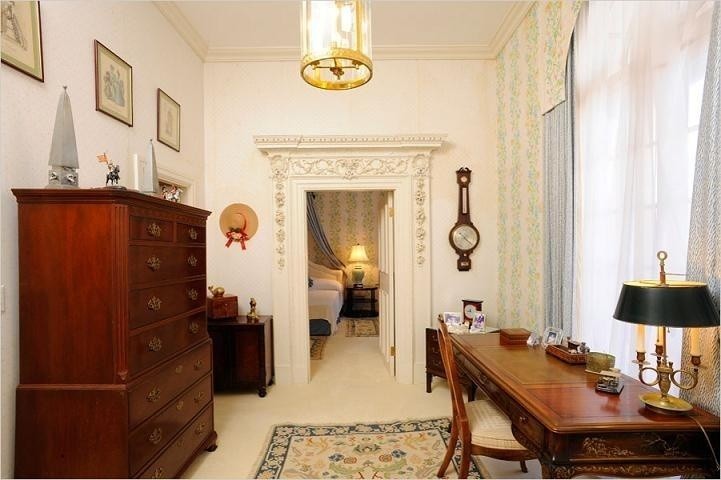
[437,312,539,479]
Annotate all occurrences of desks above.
[449,332,721,479]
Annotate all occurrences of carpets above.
[247,416,492,479]
[343,317,379,337]
[310,336,329,361]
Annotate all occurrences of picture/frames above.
[0,1,46,82]
[94,38,135,130]
[157,86,182,155]
[541,327,564,349]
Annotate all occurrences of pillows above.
[309,275,318,290]
[317,279,340,291]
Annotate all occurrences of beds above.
[308,260,346,336]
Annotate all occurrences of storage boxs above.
[208,293,239,320]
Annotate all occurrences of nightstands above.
[343,286,379,318]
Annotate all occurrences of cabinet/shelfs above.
[11,186,218,478]
[208,315,275,398]
[426,327,478,403]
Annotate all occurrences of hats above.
[220,203,259,242]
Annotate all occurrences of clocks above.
[461,299,483,329]
[448,166,481,271]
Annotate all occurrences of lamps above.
[613,252,721,417]
[299,1,376,92]
[347,242,370,288]
